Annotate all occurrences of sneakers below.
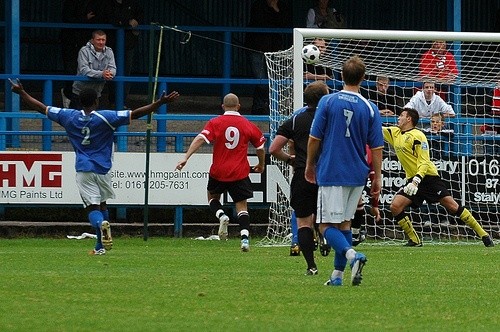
[349,252,367,286]
[319,233,331,256]
[323,278,341,286]
[403,239,422,247]
[314,236,318,251]
[241,238,249,252]
[304,267,318,276]
[290,243,299,256]
[352,230,365,246]
[218,215,229,240]
[88,248,105,255]
[100,221,113,250]
[482,235,494,247]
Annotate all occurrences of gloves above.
[403,176,421,195]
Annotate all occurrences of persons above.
[404,81,455,118]
[424,114,453,161]
[269,80,331,276]
[290,209,319,256]
[62,0,143,74]
[69,30,117,110]
[307,0,344,29]
[251,0,286,115]
[176,93,266,251]
[8,77,180,255]
[420,40,460,100]
[303,38,332,80]
[305,56,384,287]
[381,108,494,248]
[369,76,404,117]
[481,86,500,163]
[350,144,381,247]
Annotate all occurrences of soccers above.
[301,44,321,64]
